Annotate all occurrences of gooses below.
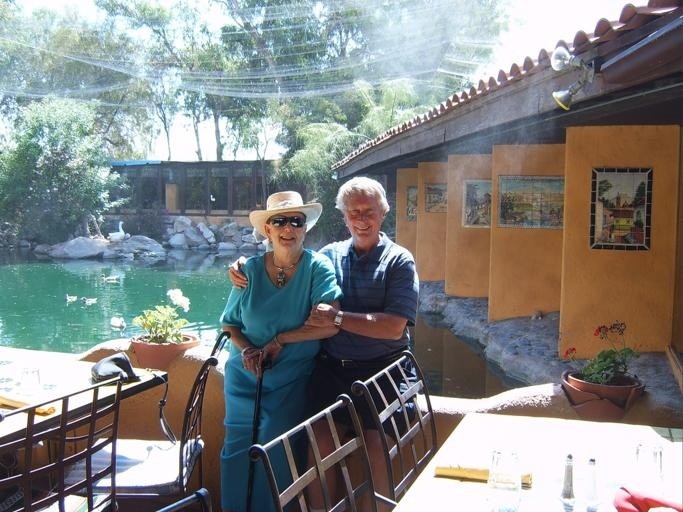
[108,220,126,243]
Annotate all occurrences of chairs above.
[247,348,438,512]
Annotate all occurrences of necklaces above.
[270,255,299,286]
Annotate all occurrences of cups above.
[485,448,523,512]
[636,443,665,495]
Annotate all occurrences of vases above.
[559,371,645,421]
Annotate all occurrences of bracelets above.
[240,346,256,356]
[333,310,344,327]
[273,335,283,350]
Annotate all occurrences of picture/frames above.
[406,183,448,224]
[496,176,563,230]
[462,179,492,229]
[588,168,652,251]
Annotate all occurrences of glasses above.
[271,217,306,228]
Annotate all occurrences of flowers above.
[564,320,643,385]
[131,288,190,343]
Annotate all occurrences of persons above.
[223,174,419,512]
[217,187,344,512]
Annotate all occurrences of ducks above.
[99,274,120,284]
[64,293,77,302]
[110,316,126,328]
[80,297,97,303]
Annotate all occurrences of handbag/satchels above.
[92,350,139,385]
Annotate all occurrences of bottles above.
[584,458,601,512]
[558,453,576,504]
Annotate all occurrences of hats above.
[249,191,323,239]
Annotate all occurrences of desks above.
[392,413,683,511]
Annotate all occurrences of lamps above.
[549,46,598,111]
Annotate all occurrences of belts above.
[322,346,409,368]
